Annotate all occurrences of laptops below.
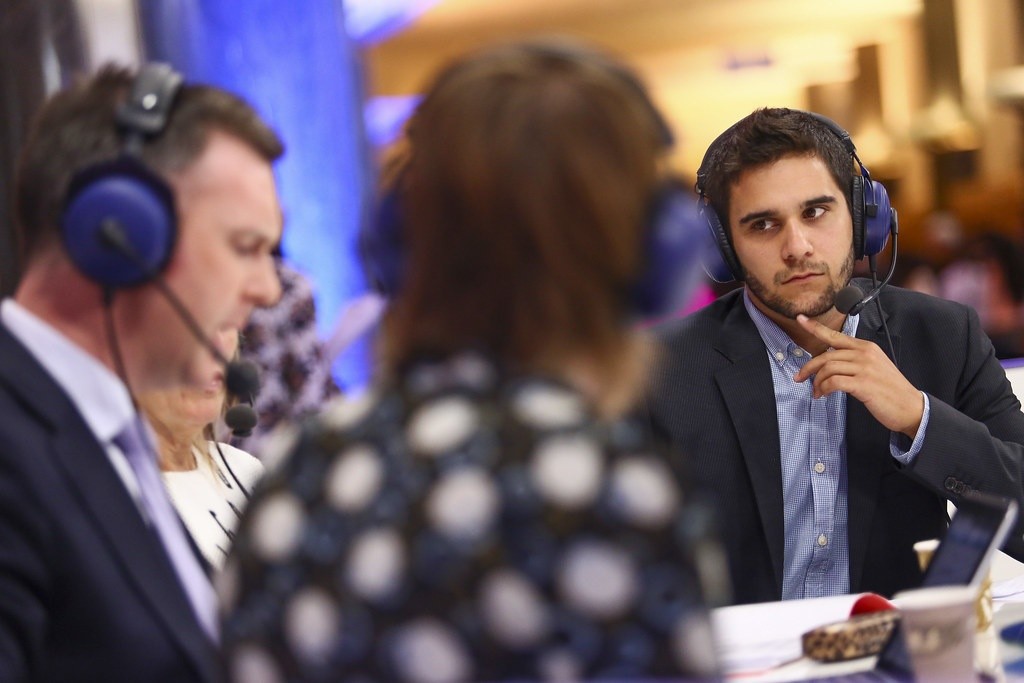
[783,490,1020,683]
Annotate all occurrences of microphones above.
[156,276,263,437]
[834,234,899,316]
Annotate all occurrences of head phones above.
[693,110,899,284]
[51,62,185,296]
[356,44,708,326]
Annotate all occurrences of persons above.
[135,347,266,584]
[624,105,1024,604]
[213,36,721,683]
[0,59,285,683]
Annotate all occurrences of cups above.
[912,539,993,635]
[894,584,977,682]
[983,575,1024,683]
